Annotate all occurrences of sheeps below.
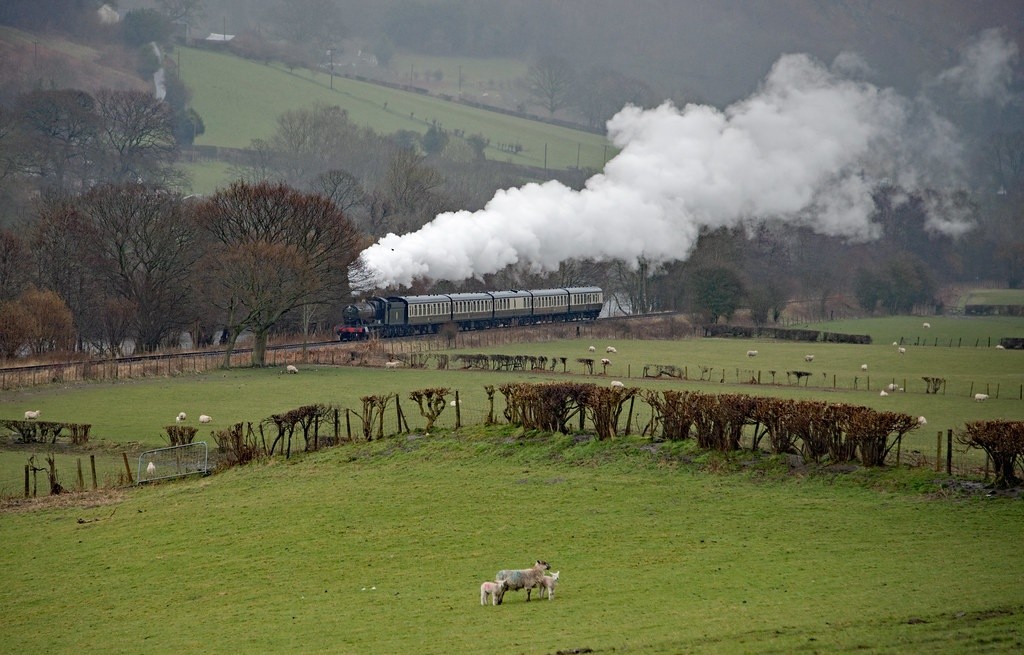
[975,393,990,403]
[147,461,156,477]
[385,360,400,372]
[199,414,213,423]
[861,340,929,426]
[747,350,758,358]
[601,358,612,367]
[995,345,1006,351]
[610,380,624,388]
[287,365,298,374]
[176,412,186,424]
[479,559,560,605]
[589,346,595,352]
[450,399,462,407]
[607,346,617,353]
[805,354,814,362]
[25,410,41,421]
[923,322,931,329]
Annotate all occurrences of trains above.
[333,285,603,343]
